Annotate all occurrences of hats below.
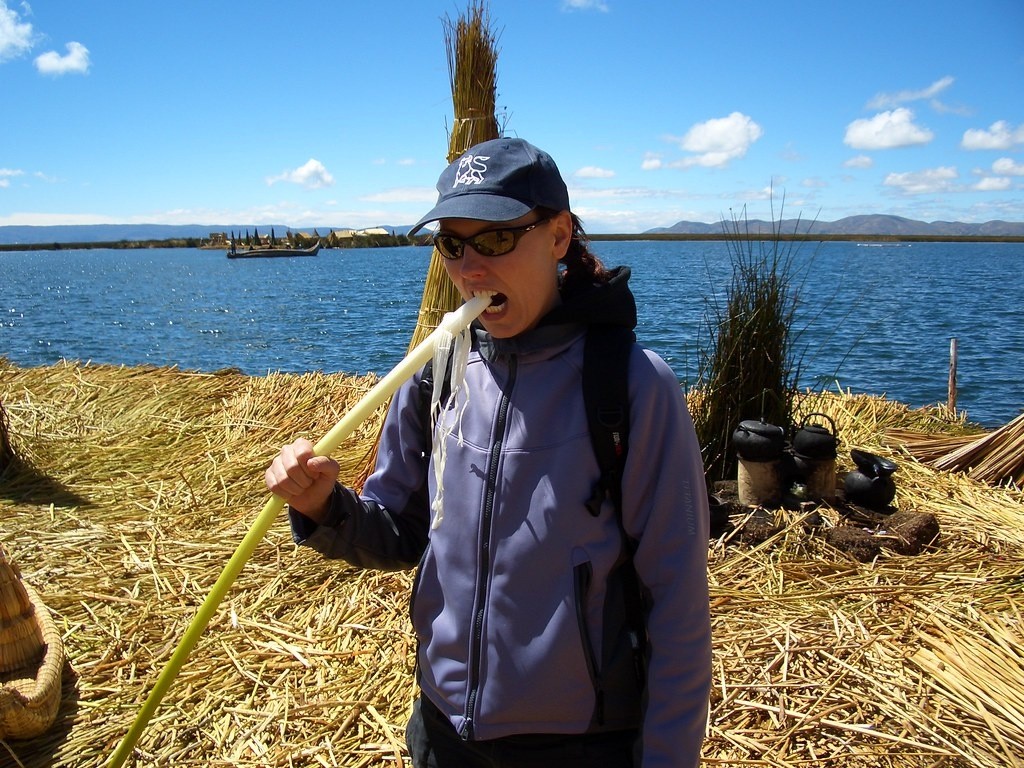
[406,137,569,239]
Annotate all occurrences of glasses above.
[433,219,551,259]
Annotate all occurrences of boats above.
[227,240,321,260]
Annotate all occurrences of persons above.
[261,139,713,768]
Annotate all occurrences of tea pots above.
[708,494,729,540]
[844,469,896,508]
[787,412,841,460]
[733,415,784,461]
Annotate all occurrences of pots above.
[850,448,898,476]
